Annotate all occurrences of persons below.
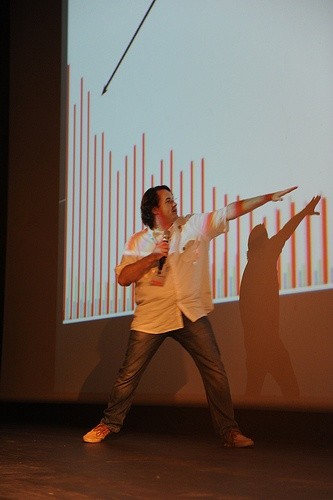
[83,185,298,447]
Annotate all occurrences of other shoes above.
[223,431,253,449]
[82,420,111,442]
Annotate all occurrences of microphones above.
[157,230,171,274]
